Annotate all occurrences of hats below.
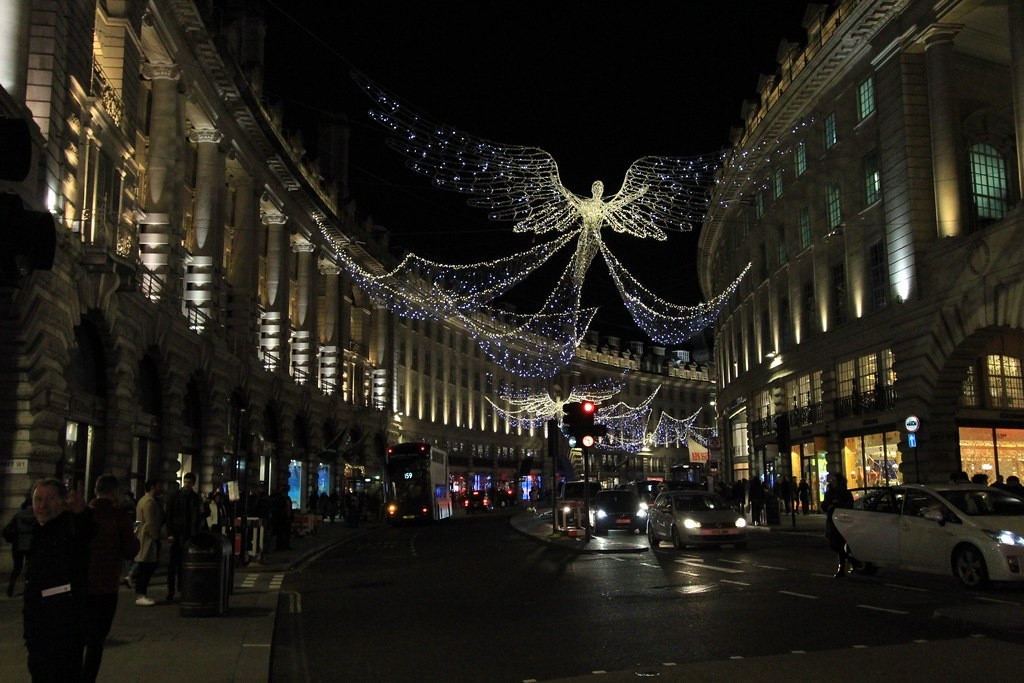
[256,481,266,486]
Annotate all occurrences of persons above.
[678,461,814,529]
[3,472,562,683]
[819,474,854,580]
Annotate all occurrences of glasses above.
[258,485,267,489]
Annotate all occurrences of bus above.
[387,443,454,525]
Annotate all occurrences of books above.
[943,470,1022,516]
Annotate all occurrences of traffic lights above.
[563,401,607,448]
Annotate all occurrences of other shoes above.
[124,575,133,589]
[157,594,177,606]
[135,596,156,607]
[848,569,865,580]
[7,583,15,596]
[831,570,846,578]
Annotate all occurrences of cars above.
[832,483,1024,588]
[555,477,707,536]
[463,488,518,513]
[646,490,749,550]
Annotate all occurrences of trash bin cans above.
[343,500,360,528]
[179,532,233,617]
